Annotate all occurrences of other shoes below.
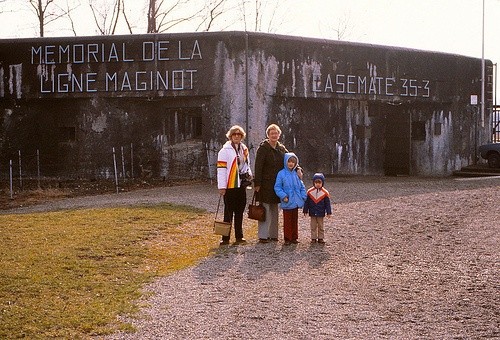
[292,239,300,244]
[220,240,230,245]
[284,240,291,245]
[259,238,271,243]
[271,238,278,241]
[236,238,246,242]
[311,239,316,243]
[318,239,325,243]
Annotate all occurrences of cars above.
[480,142,500,167]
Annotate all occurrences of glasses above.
[232,132,241,136]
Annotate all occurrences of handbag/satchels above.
[248,191,266,221]
[212,220,231,236]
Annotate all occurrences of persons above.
[303,173,332,243]
[275,153,307,246]
[254,124,304,243]
[217,125,253,245]
